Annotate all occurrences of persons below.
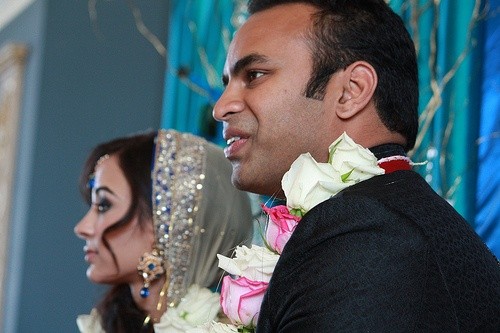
[74,128,254,333]
[212,0,500,333]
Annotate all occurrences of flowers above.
[76,306,106,333]
[152,130,387,333]
